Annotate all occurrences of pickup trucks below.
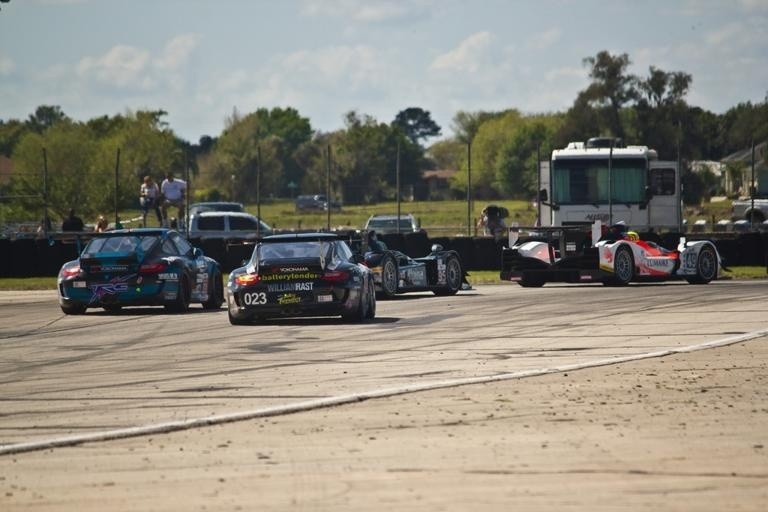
[729,196,767,221]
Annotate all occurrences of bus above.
[538,137,683,232]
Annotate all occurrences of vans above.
[191,212,275,230]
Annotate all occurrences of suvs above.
[187,201,243,226]
[294,195,341,215]
[365,212,420,233]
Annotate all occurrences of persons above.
[62,207,84,232]
[613,220,639,240]
[477,204,510,236]
[138,175,162,228]
[37,215,52,232]
[159,171,190,227]
[116,214,125,230]
[94,214,109,232]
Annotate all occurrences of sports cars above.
[498,220,720,289]
[346,230,467,298]
[223,231,381,325]
[55,228,222,314]
